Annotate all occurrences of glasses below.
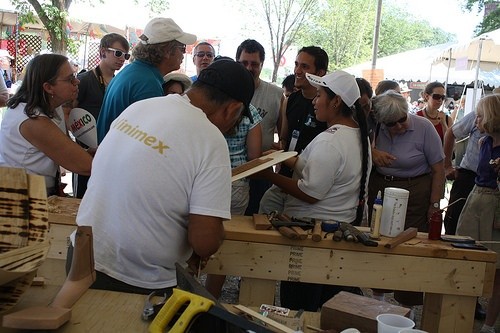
[382,112,407,127]
[194,51,215,58]
[428,93,445,101]
[237,60,262,67]
[49,76,76,84]
[172,44,186,50]
[106,48,131,60]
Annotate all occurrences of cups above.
[377,314,415,333]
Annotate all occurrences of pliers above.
[142,291,167,320]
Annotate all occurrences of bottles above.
[379,188,410,237]
[429,212,442,239]
[368,190,382,239]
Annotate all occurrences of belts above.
[376,170,430,181]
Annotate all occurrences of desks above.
[0,194,498,333]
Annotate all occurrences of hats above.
[197,59,255,124]
[0,50,14,59]
[305,69,361,111]
[140,17,197,45]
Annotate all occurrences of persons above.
[0,17,500,327]
